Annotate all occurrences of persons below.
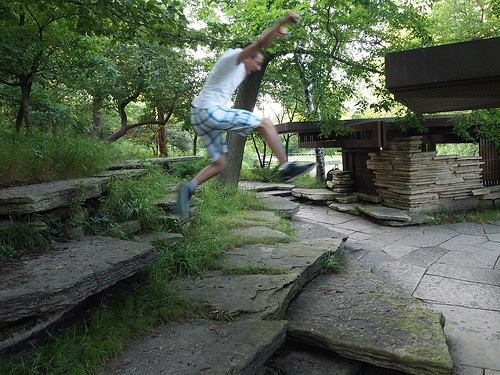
[177,12,320,217]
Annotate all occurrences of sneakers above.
[178,183,193,219]
[282,160,319,184]
